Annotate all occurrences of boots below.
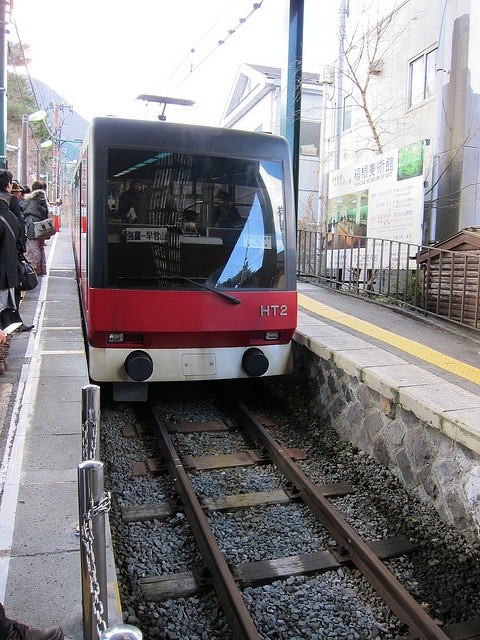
[4,335,11,370]
[0,336,6,374]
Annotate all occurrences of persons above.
[177,209,205,237]
[0,168,55,377]
[119,178,144,220]
[213,190,241,229]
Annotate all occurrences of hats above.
[11,180,23,191]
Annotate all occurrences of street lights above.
[21,110,46,186]
[37,140,52,180]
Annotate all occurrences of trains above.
[71,118,297,401]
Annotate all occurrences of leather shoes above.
[15,323,35,334]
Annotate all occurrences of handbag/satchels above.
[33,219,56,239]
[0,215,38,291]
[1,309,22,336]
